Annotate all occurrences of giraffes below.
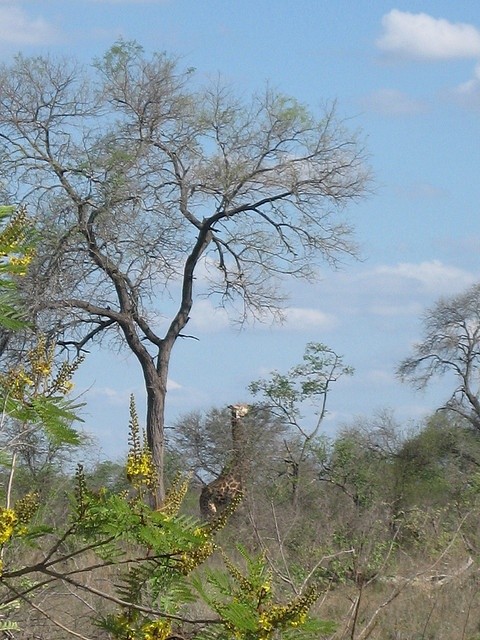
[200,404,253,521]
